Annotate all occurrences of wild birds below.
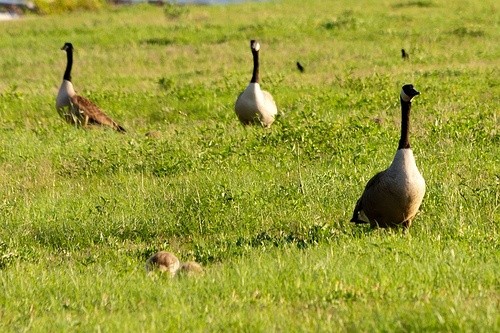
[235,40,278,126]
[56,42,127,135]
[349,85,427,235]
